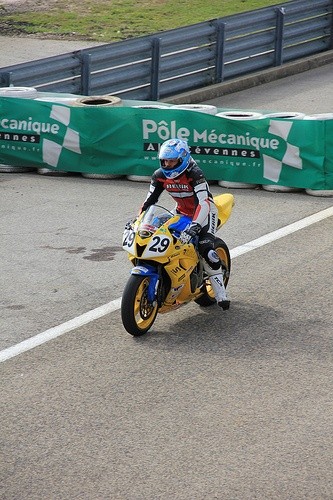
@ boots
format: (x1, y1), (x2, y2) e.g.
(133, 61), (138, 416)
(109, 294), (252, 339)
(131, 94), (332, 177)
(208, 273), (230, 311)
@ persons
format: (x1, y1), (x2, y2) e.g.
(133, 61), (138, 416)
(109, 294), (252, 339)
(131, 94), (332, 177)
(132, 138), (231, 311)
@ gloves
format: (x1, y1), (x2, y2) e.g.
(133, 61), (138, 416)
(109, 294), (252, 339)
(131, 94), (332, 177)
(180, 222), (202, 244)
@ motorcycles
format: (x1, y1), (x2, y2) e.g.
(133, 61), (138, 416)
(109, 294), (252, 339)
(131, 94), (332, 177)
(120, 193), (235, 337)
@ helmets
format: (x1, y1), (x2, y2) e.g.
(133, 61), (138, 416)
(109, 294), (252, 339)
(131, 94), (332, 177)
(158, 139), (192, 180)
